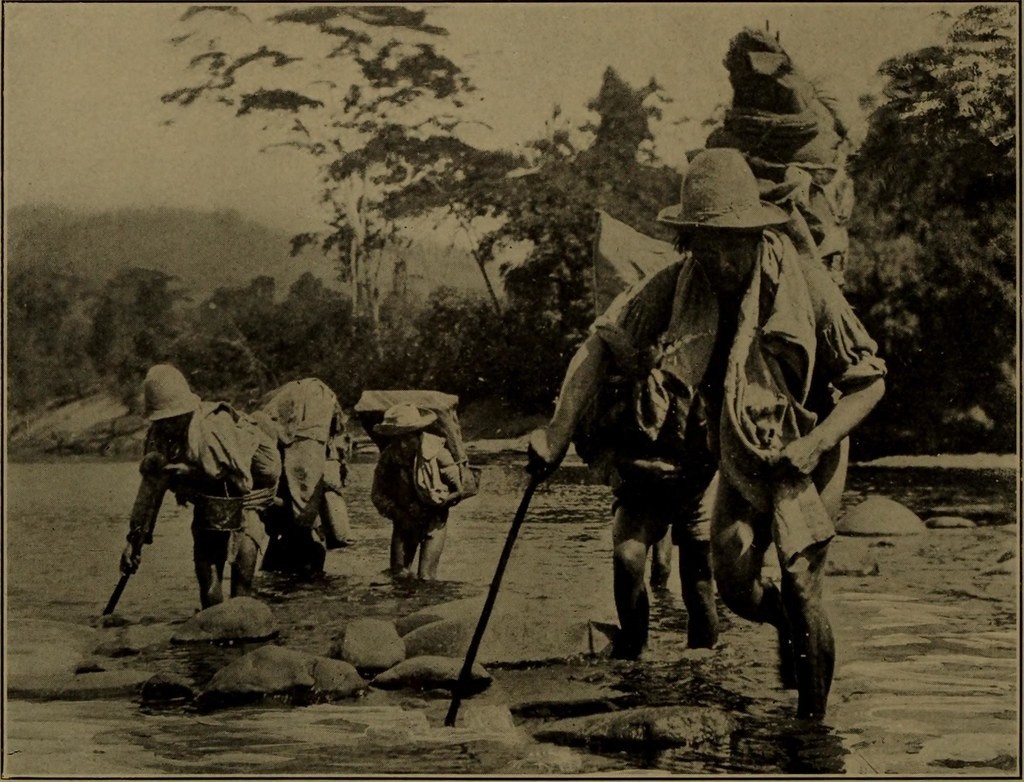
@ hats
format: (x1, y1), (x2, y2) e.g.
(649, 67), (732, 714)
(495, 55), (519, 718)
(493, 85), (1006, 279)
(655, 147), (790, 232)
(141, 364), (200, 422)
(373, 401), (438, 436)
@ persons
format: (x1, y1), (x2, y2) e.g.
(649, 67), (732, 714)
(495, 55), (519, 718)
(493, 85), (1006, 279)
(521, 25), (887, 727)
(118, 364), (477, 610)
